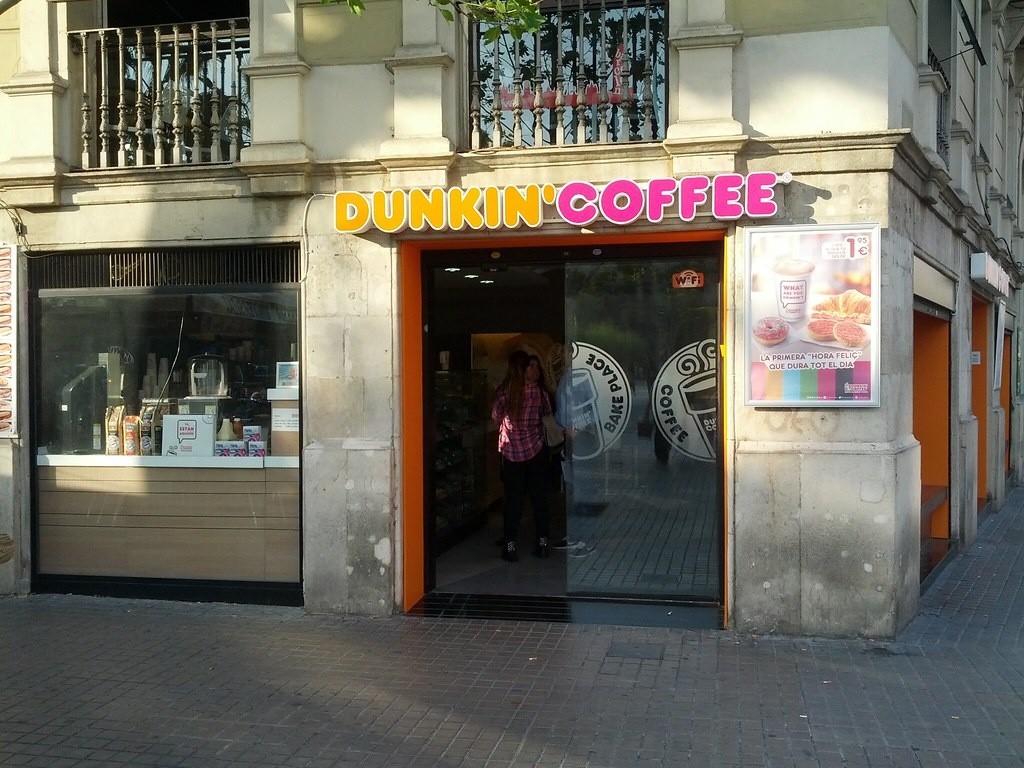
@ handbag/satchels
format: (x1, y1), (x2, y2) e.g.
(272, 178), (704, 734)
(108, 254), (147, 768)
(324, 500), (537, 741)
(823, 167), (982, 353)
(538, 387), (565, 449)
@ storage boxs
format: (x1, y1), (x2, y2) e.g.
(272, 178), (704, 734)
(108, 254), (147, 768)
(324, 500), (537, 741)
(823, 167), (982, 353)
(162, 414), (267, 457)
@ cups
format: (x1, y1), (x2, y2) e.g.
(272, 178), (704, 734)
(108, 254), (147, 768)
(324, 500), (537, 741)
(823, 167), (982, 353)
(774, 261), (815, 323)
(138, 354), (169, 397)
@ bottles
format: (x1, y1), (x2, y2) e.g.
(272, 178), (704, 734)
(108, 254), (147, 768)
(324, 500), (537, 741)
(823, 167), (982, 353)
(216, 416), (236, 441)
(234, 418), (243, 439)
(186, 352), (224, 396)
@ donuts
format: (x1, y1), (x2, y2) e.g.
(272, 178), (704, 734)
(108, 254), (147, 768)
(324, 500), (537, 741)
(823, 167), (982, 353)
(753, 316), (790, 346)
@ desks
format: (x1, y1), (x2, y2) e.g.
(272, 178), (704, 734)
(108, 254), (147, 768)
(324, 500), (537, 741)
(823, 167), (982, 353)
(751, 291), (871, 400)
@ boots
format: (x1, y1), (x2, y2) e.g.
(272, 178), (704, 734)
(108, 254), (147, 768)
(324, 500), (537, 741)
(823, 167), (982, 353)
(534, 535), (552, 559)
(501, 535), (522, 563)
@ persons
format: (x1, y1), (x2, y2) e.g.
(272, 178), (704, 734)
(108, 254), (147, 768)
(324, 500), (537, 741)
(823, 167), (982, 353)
(647, 368), (694, 468)
(490, 349), (596, 562)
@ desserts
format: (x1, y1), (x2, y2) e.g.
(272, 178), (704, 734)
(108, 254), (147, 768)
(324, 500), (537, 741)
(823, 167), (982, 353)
(833, 321), (868, 347)
(807, 319), (839, 341)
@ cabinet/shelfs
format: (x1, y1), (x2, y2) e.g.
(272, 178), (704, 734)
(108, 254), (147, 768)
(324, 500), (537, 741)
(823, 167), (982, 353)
(422, 369), (491, 556)
(268, 387), (300, 457)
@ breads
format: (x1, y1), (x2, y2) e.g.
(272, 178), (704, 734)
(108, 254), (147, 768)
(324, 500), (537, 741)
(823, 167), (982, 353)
(811, 289), (873, 324)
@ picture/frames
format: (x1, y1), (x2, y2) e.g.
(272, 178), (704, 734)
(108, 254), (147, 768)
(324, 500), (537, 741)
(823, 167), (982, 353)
(275, 361), (299, 388)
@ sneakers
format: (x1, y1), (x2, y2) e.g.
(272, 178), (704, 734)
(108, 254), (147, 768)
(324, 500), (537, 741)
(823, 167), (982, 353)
(567, 541), (598, 558)
(551, 534), (580, 549)
